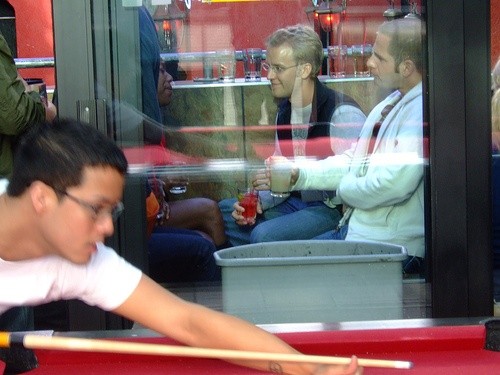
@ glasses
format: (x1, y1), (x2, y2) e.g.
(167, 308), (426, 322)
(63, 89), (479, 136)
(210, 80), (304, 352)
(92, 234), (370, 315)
(261, 62), (303, 74)
(37, 178), (125, 221)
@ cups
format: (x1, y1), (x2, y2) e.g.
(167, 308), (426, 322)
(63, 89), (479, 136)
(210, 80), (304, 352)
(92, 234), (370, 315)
(168, 160), (188, 194)
(238, 187), (258, 223)
(147, 173), (166, 218)
(217, 49), (235, 83)
(269, 162), (291, 198)
(328, 46), (347, 78)
(242, 48), (262, 81)
(351, 44), (370, 77)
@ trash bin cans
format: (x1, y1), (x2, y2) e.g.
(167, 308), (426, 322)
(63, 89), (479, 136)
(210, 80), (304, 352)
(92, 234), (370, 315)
(214, 239), (408, 325)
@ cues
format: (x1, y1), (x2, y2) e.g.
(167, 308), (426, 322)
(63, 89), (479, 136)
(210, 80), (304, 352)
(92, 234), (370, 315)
(0, 332), (412, 369)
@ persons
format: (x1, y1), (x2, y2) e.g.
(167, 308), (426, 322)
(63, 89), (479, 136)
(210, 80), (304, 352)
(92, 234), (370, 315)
(218, 23), (367, 247)
(51, 4), (232, 287)
(228, 0), (318, 126)
(0, 0), (57, 195)
(0, 118), (364, 375)
(266, 17), (423, 279)
